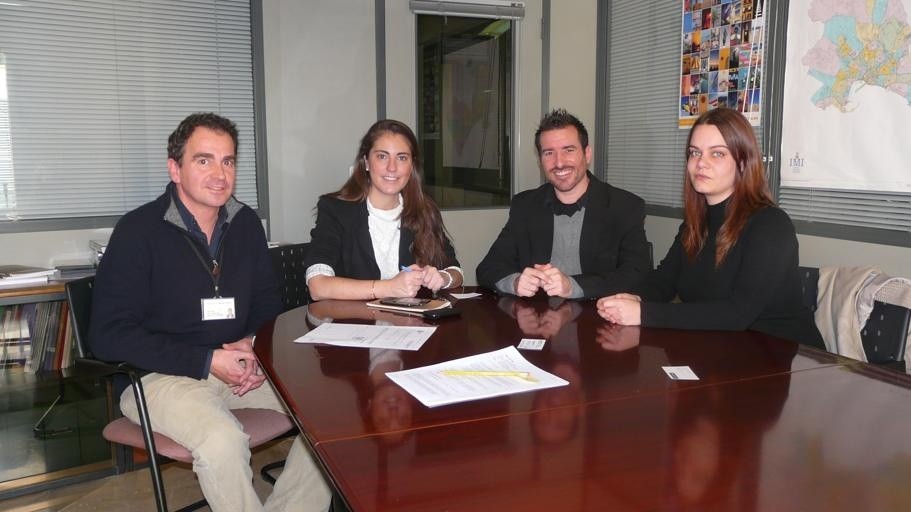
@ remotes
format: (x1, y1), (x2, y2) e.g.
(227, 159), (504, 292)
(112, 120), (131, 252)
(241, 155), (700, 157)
(422, 306), (463, 319)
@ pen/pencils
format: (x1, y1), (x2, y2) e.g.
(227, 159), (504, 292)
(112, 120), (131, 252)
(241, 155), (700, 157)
(401, 265), (413, 272)
(441, 371), (530, 376)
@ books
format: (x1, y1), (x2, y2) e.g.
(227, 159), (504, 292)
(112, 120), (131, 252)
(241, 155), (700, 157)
(1, 261), (59, 285)
(1, 298), (82, 374)
(89, 238), (110, 268)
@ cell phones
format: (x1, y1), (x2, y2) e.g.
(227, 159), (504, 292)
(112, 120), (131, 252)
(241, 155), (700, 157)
(380, 297), (431, 307)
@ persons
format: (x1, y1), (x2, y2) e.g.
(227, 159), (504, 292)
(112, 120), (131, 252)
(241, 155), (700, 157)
(87, 110), (335, 512)
(596, 108), (827, 352)
(305, 120), (466, 302)
(474, 108), (653, 303)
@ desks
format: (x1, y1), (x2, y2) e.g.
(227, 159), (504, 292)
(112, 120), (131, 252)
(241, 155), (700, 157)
(257, 279), (911, 512)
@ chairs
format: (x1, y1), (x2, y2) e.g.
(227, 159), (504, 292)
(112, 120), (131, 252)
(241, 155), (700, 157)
(798, 264), (911, 373)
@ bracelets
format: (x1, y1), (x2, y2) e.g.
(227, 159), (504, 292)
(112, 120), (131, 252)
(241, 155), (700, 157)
(439, 269), (454, 290)
(371, 279), (379, 300)
